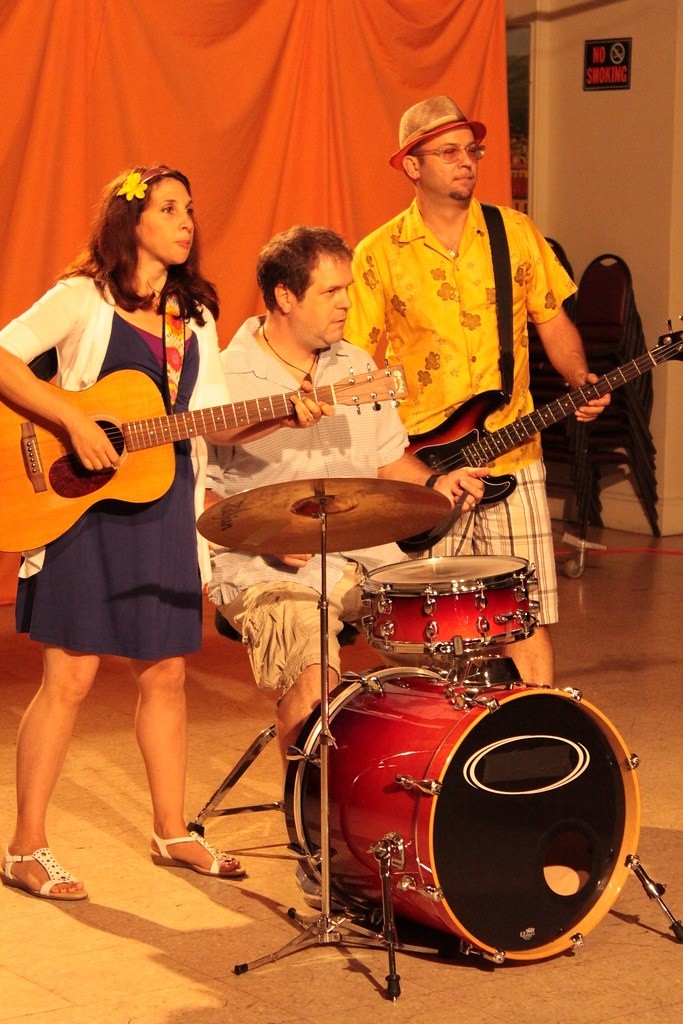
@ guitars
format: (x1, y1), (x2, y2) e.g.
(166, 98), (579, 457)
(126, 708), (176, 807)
(0, 364), (411, 554)
(378, 314), (683, 550)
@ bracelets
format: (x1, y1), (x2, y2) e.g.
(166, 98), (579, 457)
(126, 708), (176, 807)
(425, 473), (447, 488)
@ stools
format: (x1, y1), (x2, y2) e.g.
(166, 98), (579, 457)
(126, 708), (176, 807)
(187, 611), (358, 838)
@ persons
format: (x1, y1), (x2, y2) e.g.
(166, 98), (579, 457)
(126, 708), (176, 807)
(0, 163), (334, 902)
(205, 224), (490, 911)
(341, 95), (612, 689)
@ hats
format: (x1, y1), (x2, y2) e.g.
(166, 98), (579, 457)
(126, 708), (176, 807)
(389, 95), (487, 171)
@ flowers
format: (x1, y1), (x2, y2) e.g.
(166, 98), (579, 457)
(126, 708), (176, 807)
(115, 172), (148, 202)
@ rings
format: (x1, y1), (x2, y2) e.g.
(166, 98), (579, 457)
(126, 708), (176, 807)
(306, 413), (313, 421)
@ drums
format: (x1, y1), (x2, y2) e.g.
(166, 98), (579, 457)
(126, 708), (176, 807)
(364, 555), (542, 654)
(280, 664), (643, 962)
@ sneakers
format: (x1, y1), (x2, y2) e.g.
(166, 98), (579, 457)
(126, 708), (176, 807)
(295, 863), (344, 910)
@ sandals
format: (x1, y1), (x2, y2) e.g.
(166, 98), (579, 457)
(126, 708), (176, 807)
(150, 828), (246, 878)
(0, 846), (88, 901)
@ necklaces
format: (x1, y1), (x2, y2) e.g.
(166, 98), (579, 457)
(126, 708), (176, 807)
(426, 225), (462, 258)
(261, 325), (319, 385)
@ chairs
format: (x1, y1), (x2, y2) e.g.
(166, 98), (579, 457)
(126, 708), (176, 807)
(530, 238), (665, 542)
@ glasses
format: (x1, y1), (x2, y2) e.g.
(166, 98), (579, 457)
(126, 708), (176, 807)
(409, 145), (486, 164)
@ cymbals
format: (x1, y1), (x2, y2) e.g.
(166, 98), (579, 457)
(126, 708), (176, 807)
(195, 479), (453, 553)
